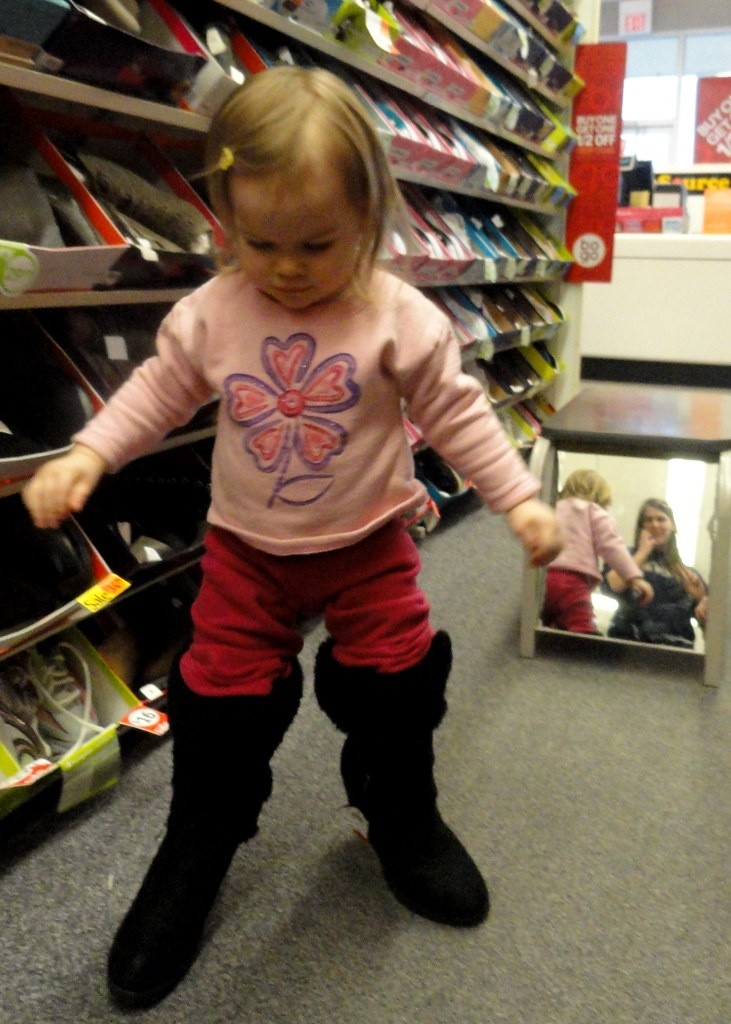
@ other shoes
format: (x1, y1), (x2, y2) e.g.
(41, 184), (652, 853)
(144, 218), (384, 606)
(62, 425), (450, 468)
(0, 0), (256, 789)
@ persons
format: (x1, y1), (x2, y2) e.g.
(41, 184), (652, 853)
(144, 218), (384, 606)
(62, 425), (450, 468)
(544, 467), (656, 635)
(601, 496), (711, 652)
(19, 68), (562, 1015)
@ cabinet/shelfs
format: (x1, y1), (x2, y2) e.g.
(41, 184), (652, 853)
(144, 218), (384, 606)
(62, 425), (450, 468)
(0, 0), (590, 856)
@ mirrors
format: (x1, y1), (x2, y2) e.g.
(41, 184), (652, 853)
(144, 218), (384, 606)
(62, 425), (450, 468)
(517, 427), (731, 687)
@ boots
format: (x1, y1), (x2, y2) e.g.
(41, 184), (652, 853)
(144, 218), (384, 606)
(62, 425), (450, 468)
(316, 623), (489, 927)
(108, 649), (304, 1016)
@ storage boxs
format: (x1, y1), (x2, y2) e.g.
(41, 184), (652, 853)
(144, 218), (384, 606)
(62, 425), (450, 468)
(2, 0), (580, 816)
